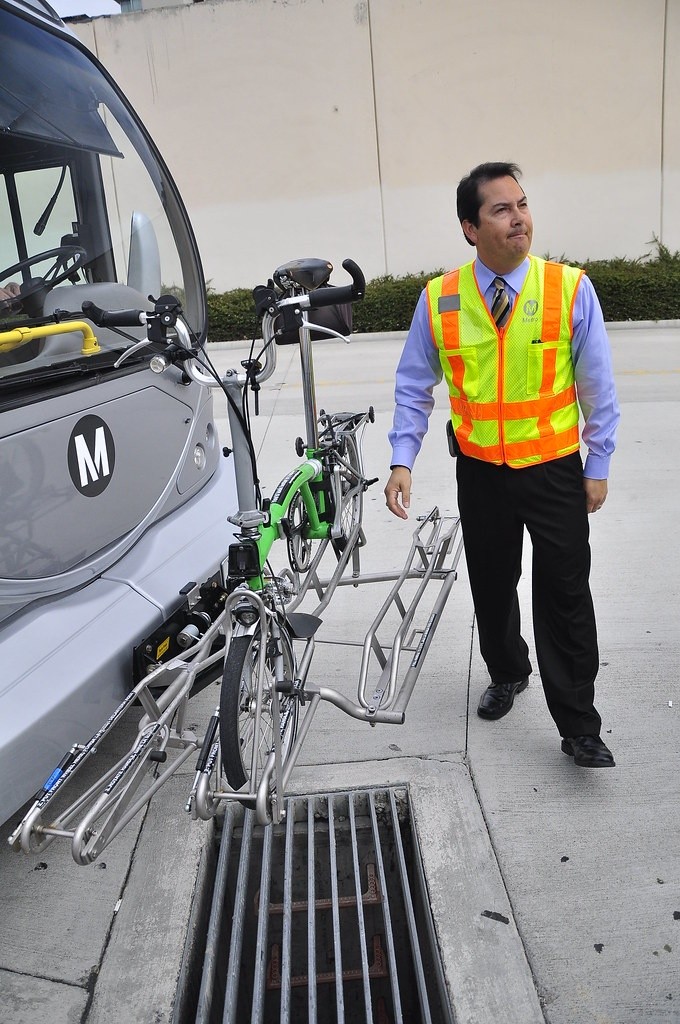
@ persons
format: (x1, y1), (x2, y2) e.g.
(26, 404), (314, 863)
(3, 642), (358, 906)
(385, 162), (616, 769)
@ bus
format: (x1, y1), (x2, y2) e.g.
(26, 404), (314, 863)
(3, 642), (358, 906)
(0, 0), (242, 830)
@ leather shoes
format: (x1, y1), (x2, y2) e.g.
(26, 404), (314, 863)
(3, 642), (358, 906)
(561, 735), (616, 767)
(477, 676), (528, 720)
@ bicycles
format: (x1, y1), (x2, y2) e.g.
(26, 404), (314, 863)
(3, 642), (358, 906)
(80, 257), (381, 813)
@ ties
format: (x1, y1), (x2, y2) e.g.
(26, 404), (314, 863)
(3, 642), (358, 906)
(492, 276), (512, 332)
